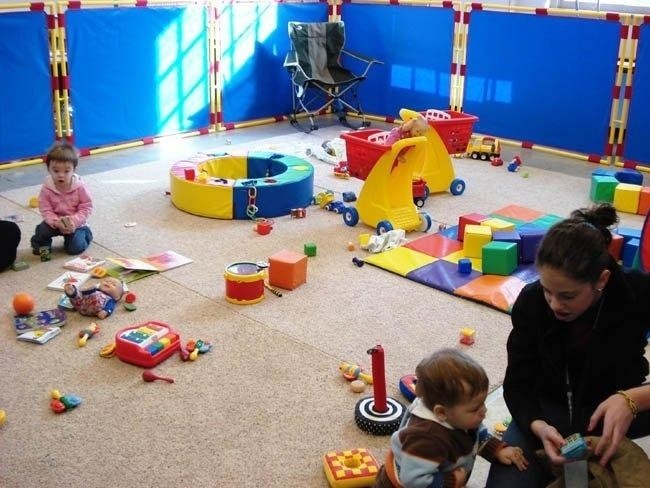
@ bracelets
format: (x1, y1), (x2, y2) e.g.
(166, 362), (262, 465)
(618, 389), (638, 418)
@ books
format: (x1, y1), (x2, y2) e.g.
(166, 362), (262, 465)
(107, 250), (191, 286)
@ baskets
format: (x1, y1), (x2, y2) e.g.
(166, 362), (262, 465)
(340, 129), (407, 181)
(418, 110), (479, 154)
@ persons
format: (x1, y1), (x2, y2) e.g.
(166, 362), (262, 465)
(483, 201), (650, 488)
(32, 141), (93, 255)
(375, 349), (528, 488)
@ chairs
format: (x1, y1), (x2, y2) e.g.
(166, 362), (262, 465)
(283, 21), (385, 133)
